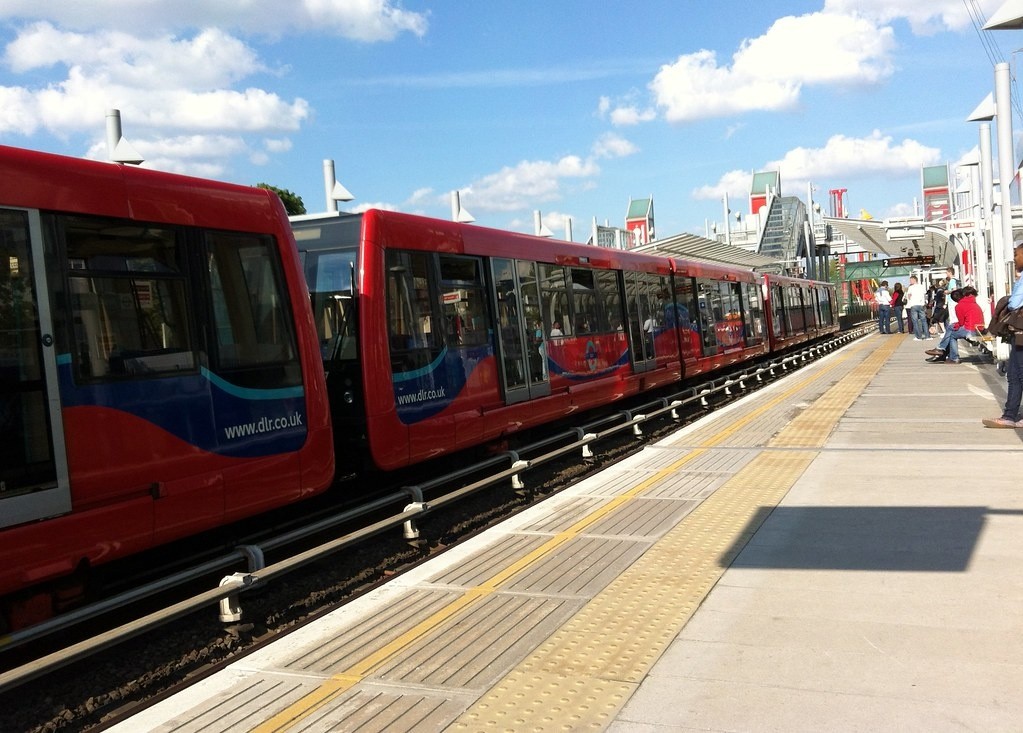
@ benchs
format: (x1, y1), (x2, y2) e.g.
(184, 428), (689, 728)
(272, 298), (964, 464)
(969, 324), (997, 357)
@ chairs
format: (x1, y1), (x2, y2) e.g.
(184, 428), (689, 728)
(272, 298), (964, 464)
(107, 344), (196, 373)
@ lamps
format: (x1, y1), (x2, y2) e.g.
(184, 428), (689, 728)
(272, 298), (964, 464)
(908, 249), (914, 256)
(900, 246), (907, 252)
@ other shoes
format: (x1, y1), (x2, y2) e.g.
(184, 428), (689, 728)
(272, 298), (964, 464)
(1014, 418), (1023, 428)
(924, 355), (946, 363)
(913, 337), (923, 341)
(926, 337), (935, 340)
(925, 347), (943, 355)
(982, 416), (1016, 429)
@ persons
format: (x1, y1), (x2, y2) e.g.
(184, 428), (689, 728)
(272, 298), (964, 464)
(535, 320), (547, 381)
(982, 242), (1023, 429)
(390, 330), (415, 372)
(890, 283), (904, 333)
(643, 311), (658, 334)
(550, 321), (563, 336)
(875, 281), (894, 334)
(908, 276), (933, 341)
(924, 286), (992, 363)
(902, 283), (913, 334)
(926, 267), (960, 338)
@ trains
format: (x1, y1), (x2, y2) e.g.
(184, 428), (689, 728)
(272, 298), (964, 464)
(0, 144), (839, 597)
